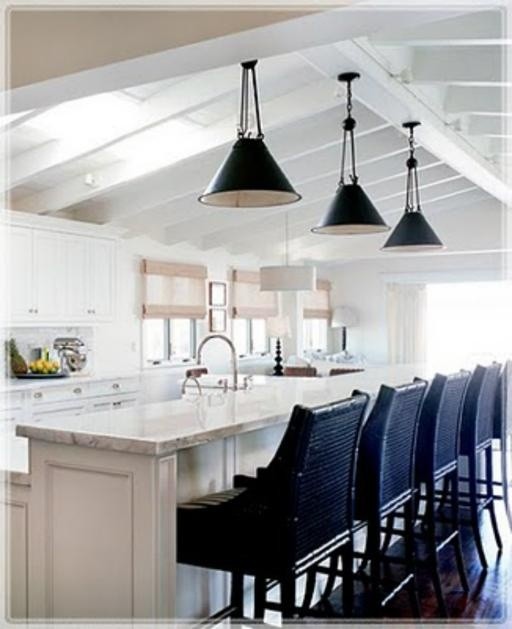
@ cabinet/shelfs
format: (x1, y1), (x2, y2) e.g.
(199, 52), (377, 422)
(27, 378), (90, 423)
(2, 207), (66, 330)
(89, 372), (143, 411)
(68, 219), (120, 327)
(1, 383), (26, 433)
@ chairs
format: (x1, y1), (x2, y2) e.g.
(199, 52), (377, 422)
(329, 368), (365, 376)
(176, 388), (369, 627)
(413, 360), (511, 569)
(284, 367), (316, 377)
(354, 369), (472, 608)
(299, 376), (430, 624)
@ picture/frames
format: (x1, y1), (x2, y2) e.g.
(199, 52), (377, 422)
(210, 308), (227, 332)
(209, 281), (226, 307)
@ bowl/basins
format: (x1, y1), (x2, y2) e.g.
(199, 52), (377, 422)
(66, 354), (87, 371)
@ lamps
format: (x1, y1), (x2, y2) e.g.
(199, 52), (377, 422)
(197, 61), (302, 208)
(266, 315), (293, 376)
(310, 72), (392, 236)
(331, 306), (360, 350)
(379, 122), (446, 254)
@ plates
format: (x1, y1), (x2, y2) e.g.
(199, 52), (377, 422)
(16, 372), (67, 380)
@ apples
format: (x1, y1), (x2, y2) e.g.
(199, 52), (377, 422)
(30, 359), (60, 374)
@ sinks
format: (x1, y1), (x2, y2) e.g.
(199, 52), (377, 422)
(185, 385), (234, 390)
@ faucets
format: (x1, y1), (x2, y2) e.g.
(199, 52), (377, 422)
(197, 334), (238, 392)
(180, 376), (203, 396)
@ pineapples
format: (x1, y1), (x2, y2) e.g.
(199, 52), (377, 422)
(9, 338), (27, 374)
